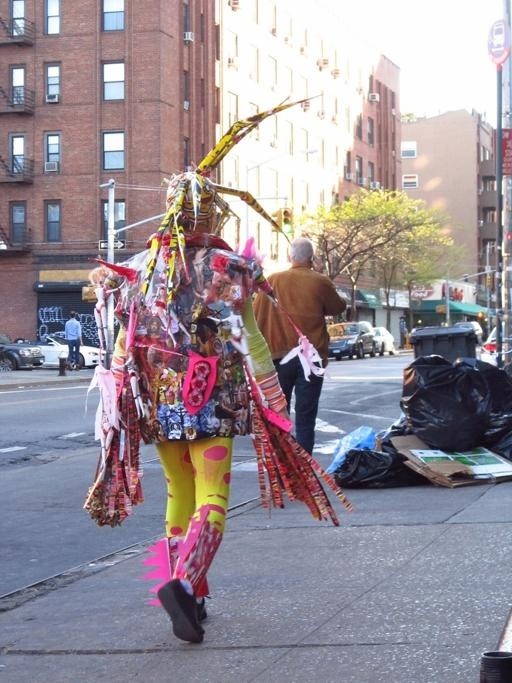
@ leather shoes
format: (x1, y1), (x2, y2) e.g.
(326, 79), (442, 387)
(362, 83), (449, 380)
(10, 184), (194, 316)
(67, 361), (81, 370)
(158, 578), (207, 642)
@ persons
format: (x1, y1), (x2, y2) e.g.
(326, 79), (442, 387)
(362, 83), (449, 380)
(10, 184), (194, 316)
(253, 237), (346, 486)
(94, 173), (347, 641)
(65, 310), (84, 372)
(399, 317), (408, 350)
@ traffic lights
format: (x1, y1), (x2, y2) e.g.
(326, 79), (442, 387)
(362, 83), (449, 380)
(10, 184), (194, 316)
(271, 208), (293, 233)
(486, 277), (490, 288)
(449, 288), (463, 301)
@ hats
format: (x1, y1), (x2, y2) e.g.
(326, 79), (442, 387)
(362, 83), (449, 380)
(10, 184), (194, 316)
(165, 171), (216, 223)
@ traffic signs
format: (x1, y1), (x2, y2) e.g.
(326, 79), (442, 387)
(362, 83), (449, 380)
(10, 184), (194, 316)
(99, 239), (126, 251)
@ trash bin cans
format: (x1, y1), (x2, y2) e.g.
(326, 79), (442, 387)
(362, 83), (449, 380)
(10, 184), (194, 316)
(410, 328), (477, 364)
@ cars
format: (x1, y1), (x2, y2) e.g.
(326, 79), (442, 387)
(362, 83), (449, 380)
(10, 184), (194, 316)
(454, 321), (511, 365)
(327, 321), (394, 360)
(0, 335), (106, 372)
